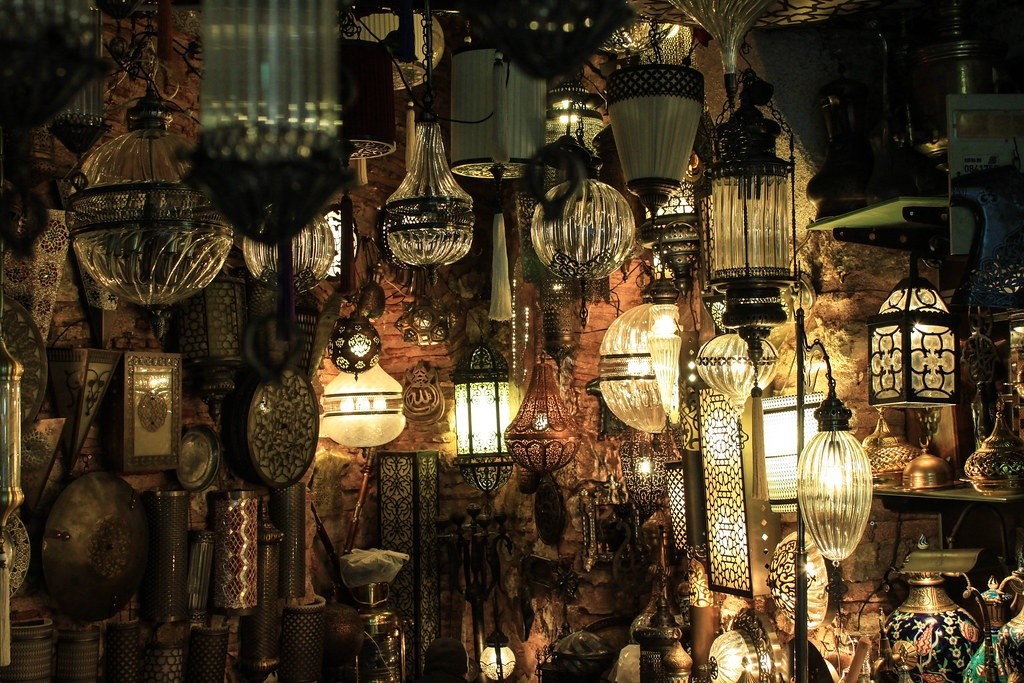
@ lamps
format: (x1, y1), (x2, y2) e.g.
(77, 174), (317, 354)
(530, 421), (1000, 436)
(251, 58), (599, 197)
(0, 0), (1024, 683)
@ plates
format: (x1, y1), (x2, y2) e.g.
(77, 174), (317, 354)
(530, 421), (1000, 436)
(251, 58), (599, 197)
(176, 423), (218, 491)
